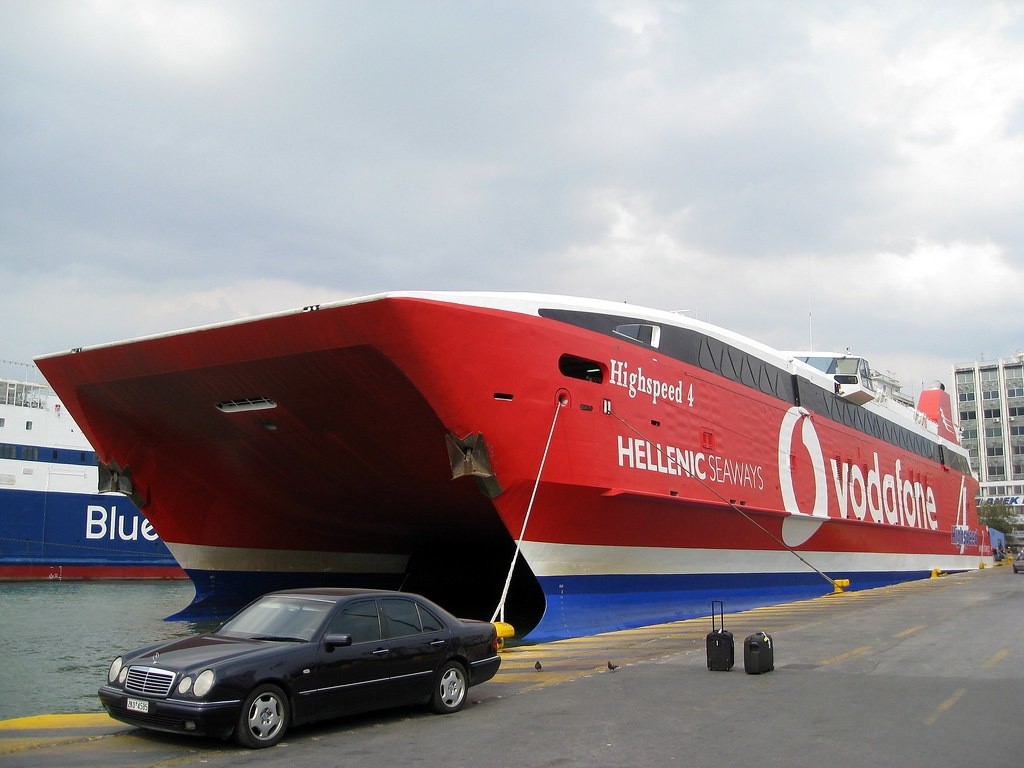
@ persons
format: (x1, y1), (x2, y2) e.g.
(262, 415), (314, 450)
(993, 544), (1013, 561)
(835, 384), (841, 392)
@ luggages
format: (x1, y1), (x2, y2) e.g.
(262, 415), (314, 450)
(706, 601), (734, 671)
(744, 633), (774, 674)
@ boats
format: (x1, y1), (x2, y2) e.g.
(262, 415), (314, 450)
(30, 283), (1007, 650)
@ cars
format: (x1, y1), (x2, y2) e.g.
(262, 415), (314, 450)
(1012, 552), (1024, 573)
(99, 585), (503, 749)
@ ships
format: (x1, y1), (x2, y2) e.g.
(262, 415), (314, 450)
(0, 375), (193, 584)
